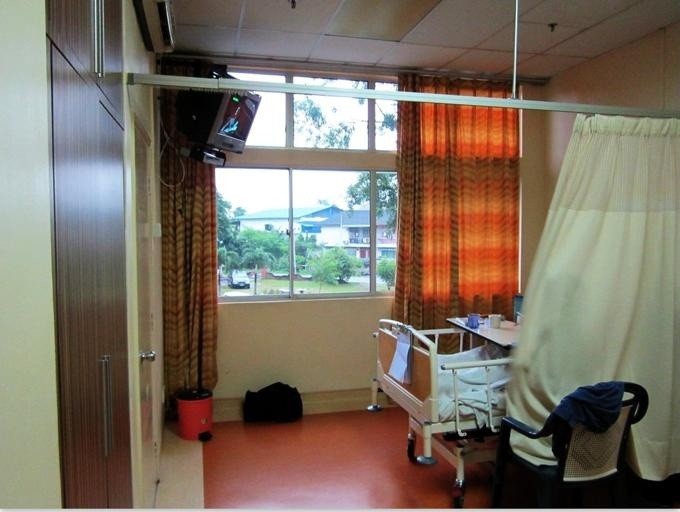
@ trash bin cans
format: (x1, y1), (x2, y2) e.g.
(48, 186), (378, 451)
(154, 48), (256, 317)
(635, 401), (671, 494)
(175, 387), (213, 440)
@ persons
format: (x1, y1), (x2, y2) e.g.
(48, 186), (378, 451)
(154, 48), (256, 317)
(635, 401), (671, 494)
(221, 107), (241, 137)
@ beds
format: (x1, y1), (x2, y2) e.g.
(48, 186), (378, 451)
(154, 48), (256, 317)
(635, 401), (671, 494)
(367, 319), (507, 491)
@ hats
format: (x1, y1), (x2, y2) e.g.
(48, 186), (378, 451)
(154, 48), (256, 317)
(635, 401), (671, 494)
(236, 106), (241, 114)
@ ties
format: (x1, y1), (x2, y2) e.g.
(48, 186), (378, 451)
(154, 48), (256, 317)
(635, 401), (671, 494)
(230, 119), (237, 127)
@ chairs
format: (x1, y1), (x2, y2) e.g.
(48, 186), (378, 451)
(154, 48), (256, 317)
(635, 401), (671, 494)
(493, 380), (649, 509)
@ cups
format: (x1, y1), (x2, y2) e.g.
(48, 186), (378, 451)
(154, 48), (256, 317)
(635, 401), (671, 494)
(467, 313), (480, 328)
(489, 315), (501, 329)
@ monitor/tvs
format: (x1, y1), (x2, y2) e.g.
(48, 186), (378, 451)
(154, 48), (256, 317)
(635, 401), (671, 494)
(175, 65), (262, 155)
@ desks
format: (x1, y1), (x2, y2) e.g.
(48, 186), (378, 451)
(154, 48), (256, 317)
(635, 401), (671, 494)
(445, 316), (520, 347)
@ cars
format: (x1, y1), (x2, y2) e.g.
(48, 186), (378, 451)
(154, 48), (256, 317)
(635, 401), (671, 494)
(227, 270), (251, 289)
